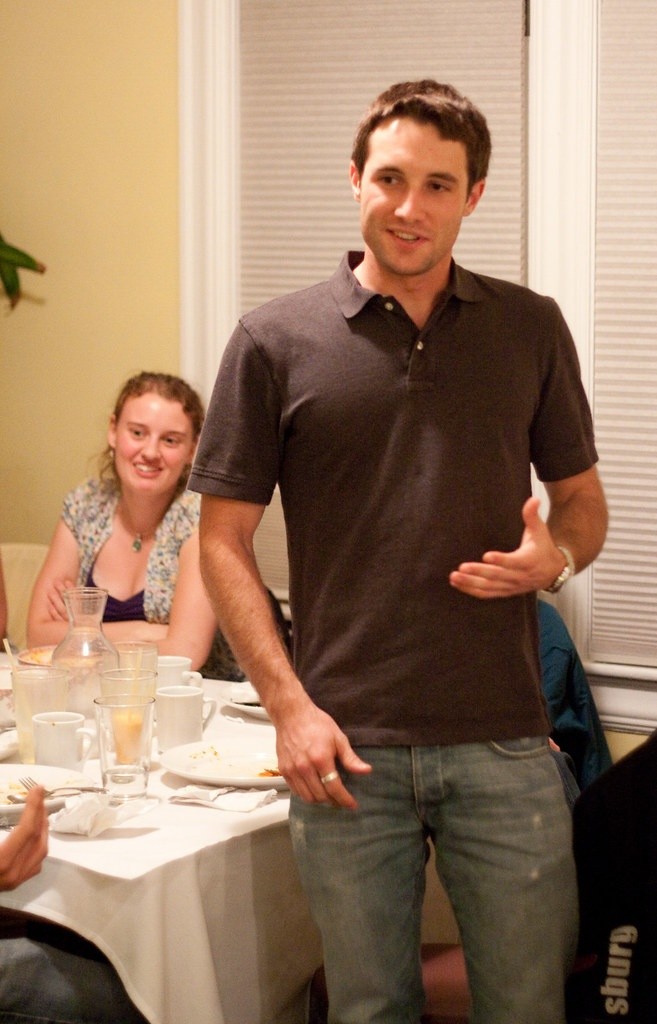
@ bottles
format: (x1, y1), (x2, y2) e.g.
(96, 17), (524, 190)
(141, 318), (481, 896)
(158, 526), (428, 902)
(52, 587), (120, 759)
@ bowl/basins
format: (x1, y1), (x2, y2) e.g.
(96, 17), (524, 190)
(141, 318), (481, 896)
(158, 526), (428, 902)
(17, 645), (58, 666)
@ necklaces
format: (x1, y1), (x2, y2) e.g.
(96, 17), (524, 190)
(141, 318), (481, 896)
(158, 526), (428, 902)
(132, 531), (148, 552)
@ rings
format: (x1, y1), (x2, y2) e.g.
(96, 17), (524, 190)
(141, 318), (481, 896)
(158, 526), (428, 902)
(321, 770), (338, 784)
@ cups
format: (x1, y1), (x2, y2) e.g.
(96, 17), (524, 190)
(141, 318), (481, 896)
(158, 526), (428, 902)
(100, 669), (157, 696)
(32, 712), (98, 770)
(10, 667), (69, 763)
(94, 695), (155, 796)
(156, 686), (217, 751)
(112, 640), (158, 670)
(157, 654), (203, 688)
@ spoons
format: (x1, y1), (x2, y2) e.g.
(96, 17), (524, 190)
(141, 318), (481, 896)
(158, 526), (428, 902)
(6, 792), (81, 804)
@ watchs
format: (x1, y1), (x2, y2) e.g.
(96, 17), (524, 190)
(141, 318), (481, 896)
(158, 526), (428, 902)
(541, 546), (575, 595)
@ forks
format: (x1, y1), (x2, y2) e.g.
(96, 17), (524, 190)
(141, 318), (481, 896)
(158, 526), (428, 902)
(19, 776), (107, 794)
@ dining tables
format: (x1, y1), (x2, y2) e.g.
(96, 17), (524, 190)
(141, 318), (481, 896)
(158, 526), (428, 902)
(1, 648), (324, 1024)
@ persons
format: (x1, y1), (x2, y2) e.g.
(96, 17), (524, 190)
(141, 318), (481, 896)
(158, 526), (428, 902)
(25, 372), (245, 682)
(0, 784), (149, 1024)
(186, 80), (610, 1024)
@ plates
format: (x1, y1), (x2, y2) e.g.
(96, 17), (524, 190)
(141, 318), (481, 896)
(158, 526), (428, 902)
(158, 738), (289, 792)
(0, 763), (95, 826)
(219, 686), (271, 721)
